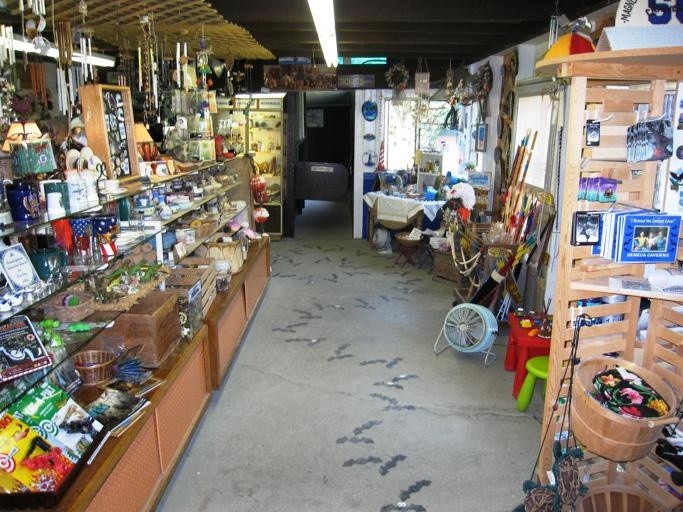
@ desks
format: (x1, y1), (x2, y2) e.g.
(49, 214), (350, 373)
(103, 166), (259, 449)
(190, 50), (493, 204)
(362, 189), (453, 250)
(504, 311), (553, 400)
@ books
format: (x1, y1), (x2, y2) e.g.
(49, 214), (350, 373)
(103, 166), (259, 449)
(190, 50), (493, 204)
(109, 376), (167, 396)
(83, 386), (151, 435)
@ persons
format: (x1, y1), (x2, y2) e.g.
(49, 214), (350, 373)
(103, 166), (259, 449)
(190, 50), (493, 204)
(633, 232), (646, 250)
(647, 233), (655, 249)
(655, 231), (666, 250)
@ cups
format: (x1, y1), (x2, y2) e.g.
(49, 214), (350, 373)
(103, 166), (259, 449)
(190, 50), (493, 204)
(69, 216), (93, 259)
(39, 176), (98, 212)
(51, 218), (73, 257)
(91, 214), (117, 256)
(424, 191), (435, 201)
(27, 247), (66, 280)
(183, 228), (195, 244)
(138, 162), (153, 178)
(103, 178), (119, 192)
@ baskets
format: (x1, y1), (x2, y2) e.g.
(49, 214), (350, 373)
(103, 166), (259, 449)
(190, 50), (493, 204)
(159, 257), (216, 320)
(43, 292), (94, 322)
(72, 350), (114, 386)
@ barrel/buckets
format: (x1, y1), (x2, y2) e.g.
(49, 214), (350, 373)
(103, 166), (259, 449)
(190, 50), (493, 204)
(570, 355), (680, 462)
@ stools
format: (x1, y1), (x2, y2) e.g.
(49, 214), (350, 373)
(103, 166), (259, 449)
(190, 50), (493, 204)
(419, 239), (435, 274)
(515, 355), (549, 412)
(390, 244), (418, 268)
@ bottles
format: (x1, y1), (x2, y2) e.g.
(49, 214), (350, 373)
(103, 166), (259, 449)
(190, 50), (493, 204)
(426, 159), (439, 174)
(152, 190), (169, 220)
(4, 182), (38, 222)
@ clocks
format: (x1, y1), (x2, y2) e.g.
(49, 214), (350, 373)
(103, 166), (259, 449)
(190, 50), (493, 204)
(306, 108), (325, 128)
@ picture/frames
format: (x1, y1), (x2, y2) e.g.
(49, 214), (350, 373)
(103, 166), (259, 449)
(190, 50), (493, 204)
(467, 171), (497, 216)
(151, 162), (171, 177)
(475, 123), (488, 152)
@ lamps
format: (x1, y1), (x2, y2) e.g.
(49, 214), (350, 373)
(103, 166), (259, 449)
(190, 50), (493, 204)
(0, 0), (116, 68)
(1, 122), (42, 153)
(535, 17), (597, 74)
(134, 121), (154, 143)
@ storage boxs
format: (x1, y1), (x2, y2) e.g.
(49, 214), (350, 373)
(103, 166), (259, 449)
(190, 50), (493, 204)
(43, 256), (217, 367)
(429, 249), (463, 289)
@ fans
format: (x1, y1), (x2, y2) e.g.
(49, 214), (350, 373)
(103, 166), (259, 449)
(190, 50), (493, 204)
(434, 303), (498, 367)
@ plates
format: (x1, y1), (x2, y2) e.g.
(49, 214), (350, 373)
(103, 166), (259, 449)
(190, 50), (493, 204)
(99, 188), (127, 195)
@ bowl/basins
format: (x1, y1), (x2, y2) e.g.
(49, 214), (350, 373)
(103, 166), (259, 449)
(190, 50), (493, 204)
(394, 232), (423, 247)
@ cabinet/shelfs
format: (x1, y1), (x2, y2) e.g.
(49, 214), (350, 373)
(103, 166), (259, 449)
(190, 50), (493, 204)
(80, 84), (142, 185)
(532, 46), (683, 512)
(417, 152), (442, 193)
(211, 92), (296, 241)
(0, 154), (272, 512)
